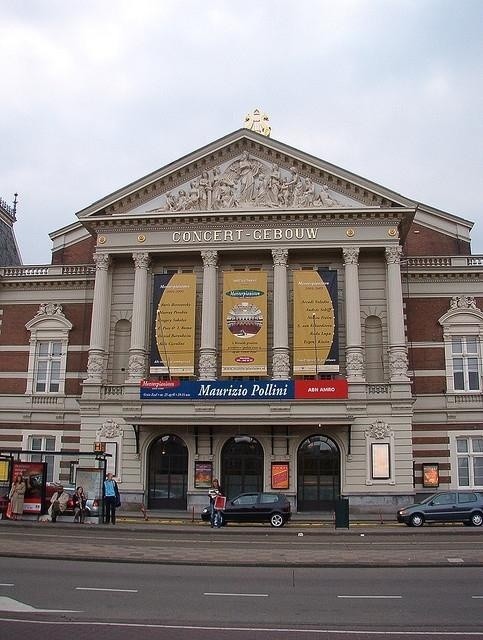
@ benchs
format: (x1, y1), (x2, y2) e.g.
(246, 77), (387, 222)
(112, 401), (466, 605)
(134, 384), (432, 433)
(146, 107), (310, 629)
(51, 510), (91, 523)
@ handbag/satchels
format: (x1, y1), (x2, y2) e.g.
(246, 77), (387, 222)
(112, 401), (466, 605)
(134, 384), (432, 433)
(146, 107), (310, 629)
(115, 494), (121, 507)
(6, 502), (13, 519)
(214, 496), (226, 510)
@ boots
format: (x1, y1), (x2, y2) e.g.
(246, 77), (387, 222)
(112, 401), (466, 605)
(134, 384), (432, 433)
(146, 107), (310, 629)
(211, 522), (221, 528)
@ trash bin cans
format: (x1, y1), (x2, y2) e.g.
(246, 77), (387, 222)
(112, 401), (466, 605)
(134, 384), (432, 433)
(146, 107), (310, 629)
(335, 499), (349, 530)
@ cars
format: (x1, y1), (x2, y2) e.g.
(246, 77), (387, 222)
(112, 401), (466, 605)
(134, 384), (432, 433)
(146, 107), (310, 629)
(150, 489), (176, 499)
(0, 482), (106, 517)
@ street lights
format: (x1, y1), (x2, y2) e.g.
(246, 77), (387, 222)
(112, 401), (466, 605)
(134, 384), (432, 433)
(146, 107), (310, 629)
(160, 449), (171, 501)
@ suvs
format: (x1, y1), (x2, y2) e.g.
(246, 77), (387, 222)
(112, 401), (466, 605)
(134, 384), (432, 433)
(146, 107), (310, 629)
(200, 490), (293, 528)
(396, 489), (482, 528)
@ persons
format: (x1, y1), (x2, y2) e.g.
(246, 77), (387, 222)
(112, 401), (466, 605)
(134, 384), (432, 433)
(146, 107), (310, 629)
(48, 485), (69, 522)
(144, 151), (336, 212)
(208, 479), (224, 528)
(8, 474), (26, 520)
(72, 487), (88, 524)
(104, 473), (118, 525)
